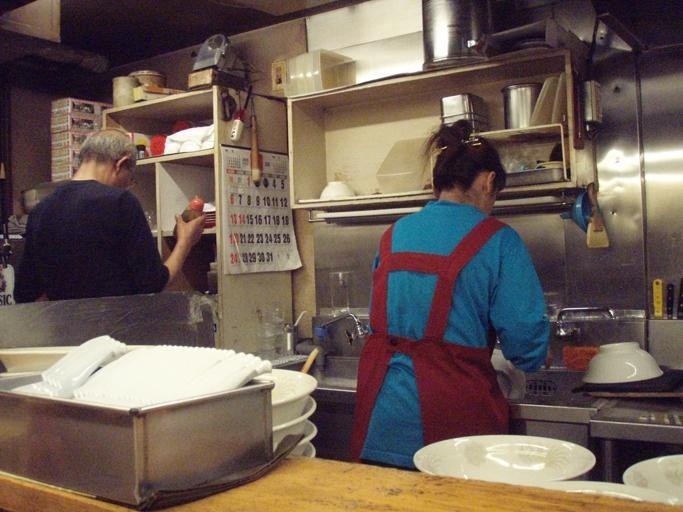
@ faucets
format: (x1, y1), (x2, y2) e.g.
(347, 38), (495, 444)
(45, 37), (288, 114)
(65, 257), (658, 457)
(282, 311), (308, 354)
(554, 304), (619, 341)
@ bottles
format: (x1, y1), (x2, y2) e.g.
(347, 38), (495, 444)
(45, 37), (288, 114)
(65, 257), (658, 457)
(136, 145), (145, 160)
(173, 196), (204, 237)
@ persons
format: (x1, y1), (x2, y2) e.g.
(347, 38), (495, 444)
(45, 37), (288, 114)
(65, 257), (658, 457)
(345, 118), (553, 474)
(12, 126), (207, 308)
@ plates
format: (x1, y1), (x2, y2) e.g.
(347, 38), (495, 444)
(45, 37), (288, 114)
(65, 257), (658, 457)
(202, 197), (217, 228)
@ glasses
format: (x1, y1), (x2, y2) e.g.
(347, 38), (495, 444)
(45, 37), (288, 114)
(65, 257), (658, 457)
(124, 164), (137, 189)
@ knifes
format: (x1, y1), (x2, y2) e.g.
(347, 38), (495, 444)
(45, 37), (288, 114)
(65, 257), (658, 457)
(653, 278), (682, 320)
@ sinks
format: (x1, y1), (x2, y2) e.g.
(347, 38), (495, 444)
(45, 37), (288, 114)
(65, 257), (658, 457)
(274, 354), (360, 391)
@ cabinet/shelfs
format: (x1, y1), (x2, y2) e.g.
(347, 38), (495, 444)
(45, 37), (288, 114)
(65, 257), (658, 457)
(287, 49), (599, 224)
(100, 85), (293, 356)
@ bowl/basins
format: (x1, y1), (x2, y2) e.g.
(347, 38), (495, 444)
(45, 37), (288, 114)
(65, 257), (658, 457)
(579, 342), (662, 386)
(319, 180), (355, 201)
(21, 187), (40, 214)
(411, 424), (683, 506)
(254, 368), (319, 456)
